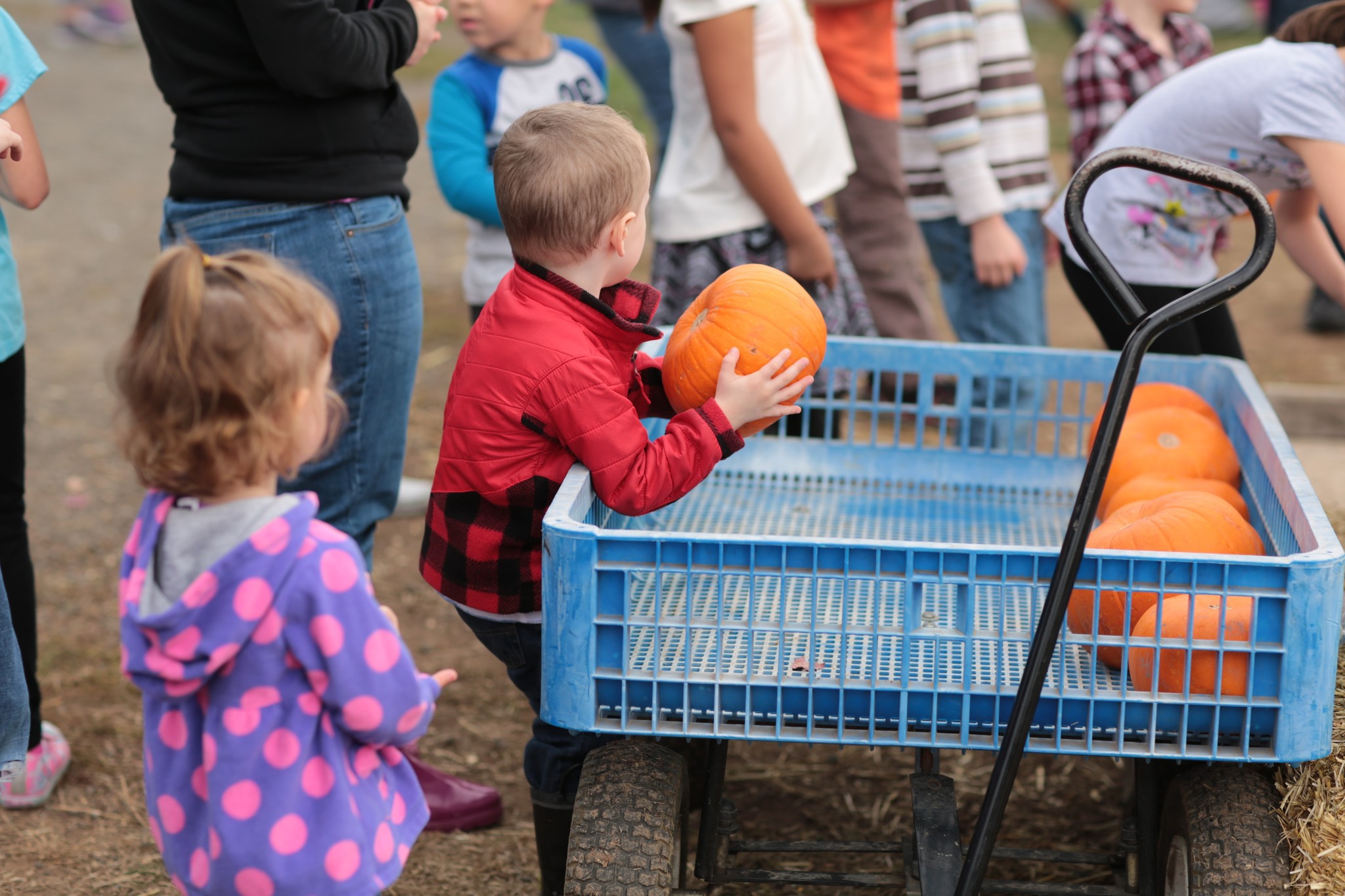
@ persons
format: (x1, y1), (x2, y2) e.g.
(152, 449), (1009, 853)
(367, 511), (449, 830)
(418, 104), (814, 896)
(1041, 0), (1345, 363)
(893, 0), (1058, 448)
(133, 0), (503, 834)
(426, 0), (610, 328)
(0, 0), (71, 810)
(120, 244), (457, 896)
(587, 0), (966, 441)
(1062, 0), (1211, 172)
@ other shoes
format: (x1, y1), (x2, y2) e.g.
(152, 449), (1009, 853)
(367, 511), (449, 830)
(393, 476), (433, 518)
(396, 752), (505, 833)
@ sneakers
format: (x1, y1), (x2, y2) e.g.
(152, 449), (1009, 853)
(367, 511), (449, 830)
(0, 719), (71, 810)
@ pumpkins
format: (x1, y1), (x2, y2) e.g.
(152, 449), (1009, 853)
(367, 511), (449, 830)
(1065, 491), (1265, 669)
(1127, 594), (1252, 696)
(1096, 407), (1240, 520)
(1103, 475), (1251, 524)
(1088, 382), (1223, 457)
(661, 264), (827, 438)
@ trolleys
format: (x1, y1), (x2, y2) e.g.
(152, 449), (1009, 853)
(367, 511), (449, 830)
(538, 141), (1345, 896)
